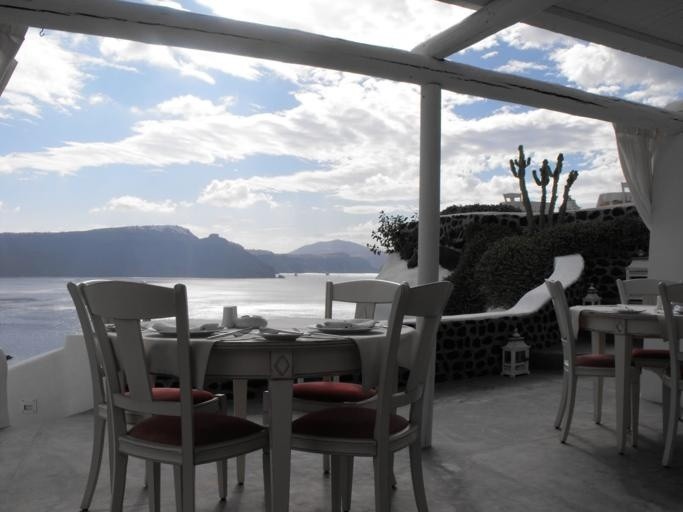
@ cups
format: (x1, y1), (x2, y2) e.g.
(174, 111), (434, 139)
(223, 306), (238, 328)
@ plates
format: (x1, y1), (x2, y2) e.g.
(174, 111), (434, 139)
(261, 332), (302, 342)
(316, 321), (371, 334)
(138, 316), (223, 336)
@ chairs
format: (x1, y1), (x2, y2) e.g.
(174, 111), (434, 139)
(546, 277), (683, 471)
(65, 278), (268, 512)
(292, 278), (455, 512)
